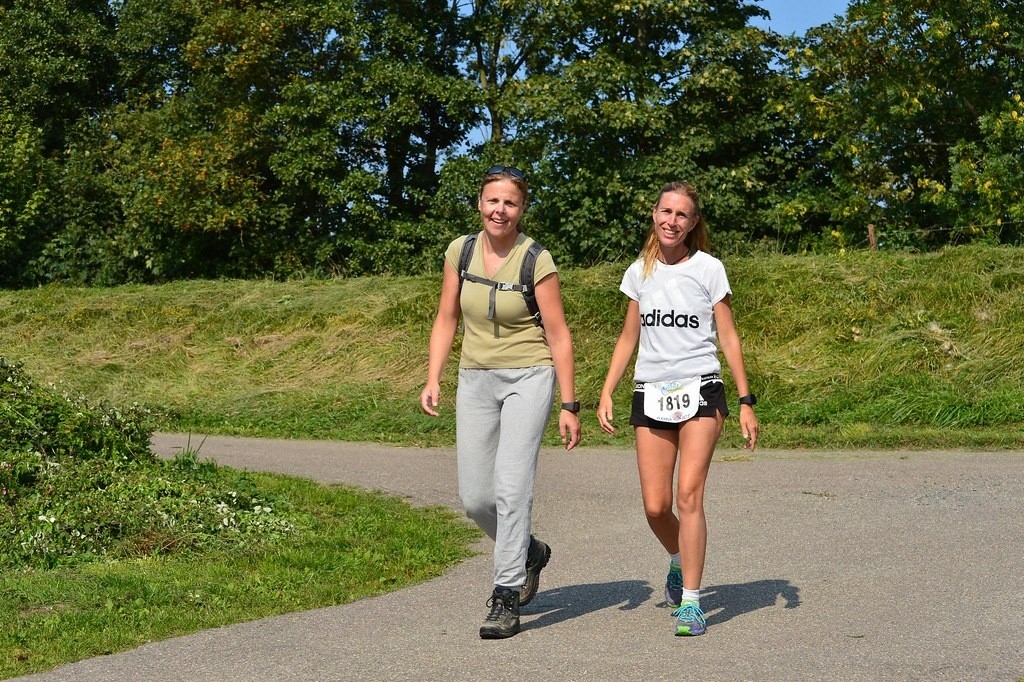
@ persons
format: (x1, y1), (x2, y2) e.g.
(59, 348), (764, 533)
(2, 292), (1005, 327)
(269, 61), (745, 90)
(596, 182), (761, 636)
(419, 166), (584, 639)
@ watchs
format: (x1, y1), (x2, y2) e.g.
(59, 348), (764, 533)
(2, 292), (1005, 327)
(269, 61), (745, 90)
(738, 394), (756, 405)
(558, 401), (580, 413)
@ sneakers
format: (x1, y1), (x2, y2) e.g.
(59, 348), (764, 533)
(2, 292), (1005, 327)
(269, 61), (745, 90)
(479, 587), (521, 637)
(672, 599), (707, 636)
(665, 560), (684, 607)
(518, 534), (551, 606)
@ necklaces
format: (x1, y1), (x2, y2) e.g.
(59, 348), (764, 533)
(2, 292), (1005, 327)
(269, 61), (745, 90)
(484, 231), (518, 269)
(658, 248), (690, 265)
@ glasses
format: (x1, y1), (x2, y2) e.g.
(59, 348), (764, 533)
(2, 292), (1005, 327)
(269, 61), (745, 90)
(485, 165), (527, 180)
